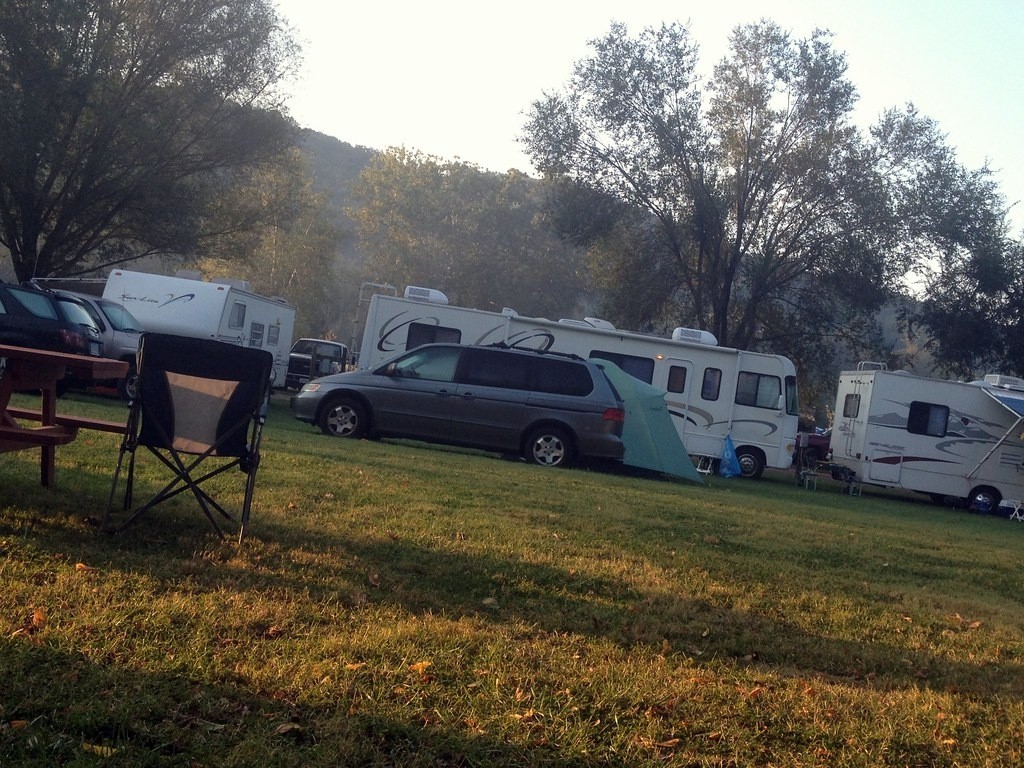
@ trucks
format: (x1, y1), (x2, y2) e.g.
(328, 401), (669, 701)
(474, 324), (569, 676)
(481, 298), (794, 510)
(357, 285), (801, 482)
(826, 363), (1023, 517)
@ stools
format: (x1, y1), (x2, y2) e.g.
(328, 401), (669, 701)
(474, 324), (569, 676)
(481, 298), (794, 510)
(1009, 499), (1024, 522)
(804, 472), (819, 490)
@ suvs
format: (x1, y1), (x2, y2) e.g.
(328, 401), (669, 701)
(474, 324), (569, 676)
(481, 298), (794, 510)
(292, 343), (628, 473)
(794, 427), (832, 470)
(49, 289), (149, 402)
(288, 337), (348, 394)
(0, 280), (106, 401)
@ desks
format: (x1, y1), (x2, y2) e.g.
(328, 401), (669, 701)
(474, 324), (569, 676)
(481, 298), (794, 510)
(0, 406), (130, 447)
(0, 344), (128, 487)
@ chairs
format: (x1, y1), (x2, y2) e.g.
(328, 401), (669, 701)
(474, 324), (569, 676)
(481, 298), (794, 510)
(305, 347), (313, 353)
(100, 332), (273, 547)
